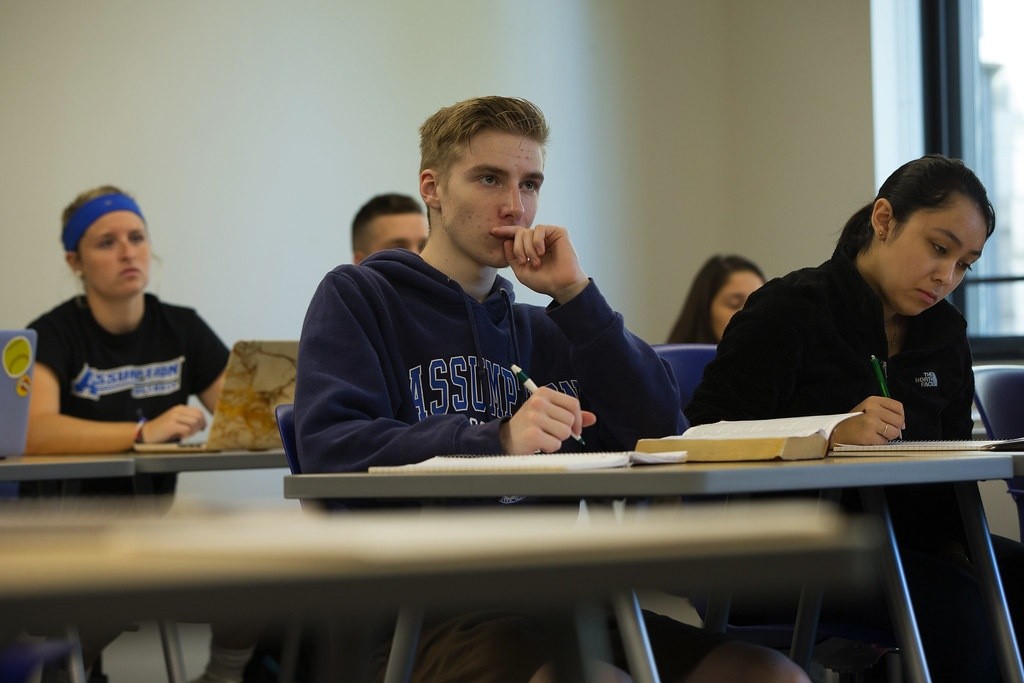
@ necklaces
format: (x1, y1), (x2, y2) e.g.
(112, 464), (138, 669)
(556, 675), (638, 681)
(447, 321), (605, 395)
(890, 314), (898, 348)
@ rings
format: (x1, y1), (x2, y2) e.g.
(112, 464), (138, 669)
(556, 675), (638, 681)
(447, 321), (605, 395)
(882, 424), (888, 436)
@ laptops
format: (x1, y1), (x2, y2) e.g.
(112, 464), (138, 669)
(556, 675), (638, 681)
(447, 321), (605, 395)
(0, 329), (37, 460)
(135, 338), (304, 453)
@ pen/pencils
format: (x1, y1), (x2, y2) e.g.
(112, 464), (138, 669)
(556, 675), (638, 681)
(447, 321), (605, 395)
(510, 363), (586, 445)
(871, 355), (904, 440)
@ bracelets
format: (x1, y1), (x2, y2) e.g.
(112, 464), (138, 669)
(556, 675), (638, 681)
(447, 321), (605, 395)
(134, 416), (147, 445)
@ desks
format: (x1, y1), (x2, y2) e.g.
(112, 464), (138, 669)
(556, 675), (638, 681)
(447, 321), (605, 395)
(0, 436), (1024, 683)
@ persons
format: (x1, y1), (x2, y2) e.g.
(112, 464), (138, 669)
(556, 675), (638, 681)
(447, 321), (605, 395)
(293, 94), (808, 680)
(664, 253), (766, 345)
(684, 155), (1023, 681)
(352, 193), (429, 266)
(20, 184), (269, 683)
